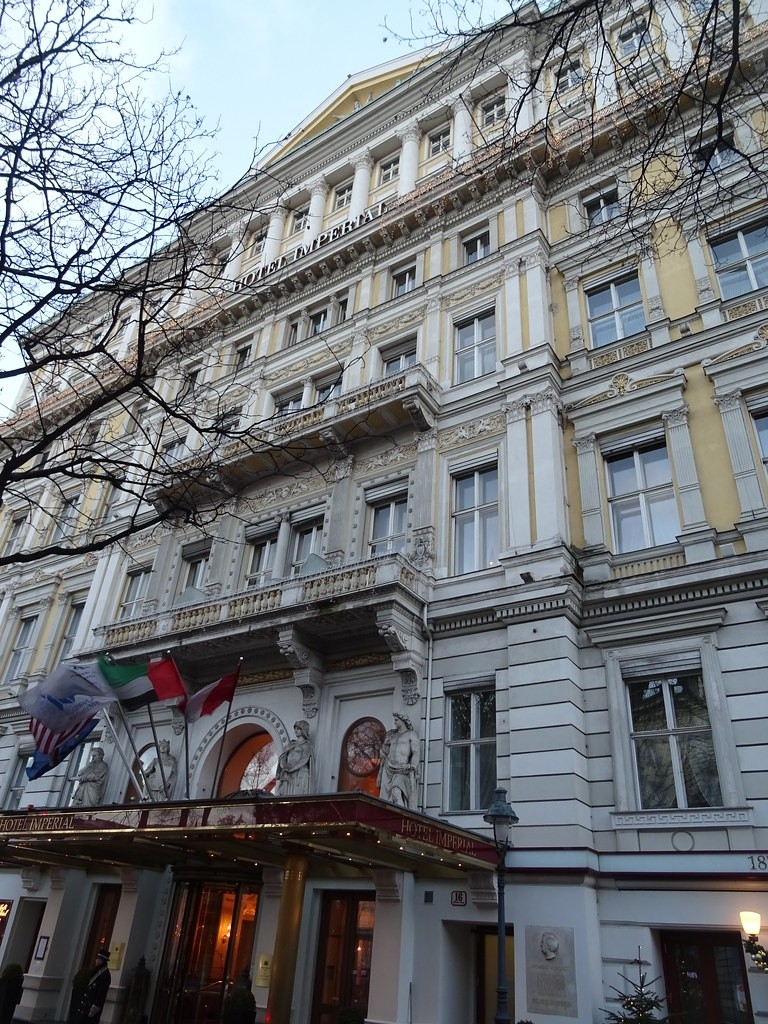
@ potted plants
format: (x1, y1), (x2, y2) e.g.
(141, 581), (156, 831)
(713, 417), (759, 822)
(222, 988), (257, 1024)
(0, 963), (24, 1024)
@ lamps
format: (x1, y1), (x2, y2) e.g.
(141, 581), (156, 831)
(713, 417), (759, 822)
(740, 909), (765, 953)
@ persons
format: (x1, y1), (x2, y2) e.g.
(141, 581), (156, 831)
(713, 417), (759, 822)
(274, 720), (317, 795)
(377, 711), (421, 808)
(141, 739), (179, 802)
(73, 949), (111, 1023)
(71, 747), (109, 807)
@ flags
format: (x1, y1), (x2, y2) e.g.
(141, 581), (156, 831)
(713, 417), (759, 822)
(17, 653), (239, 781)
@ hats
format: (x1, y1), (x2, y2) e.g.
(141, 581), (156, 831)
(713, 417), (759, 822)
(96, 949), (110, 961)
